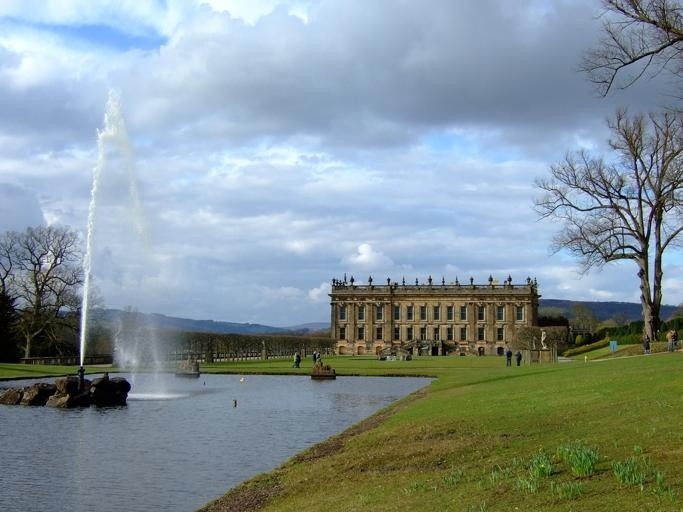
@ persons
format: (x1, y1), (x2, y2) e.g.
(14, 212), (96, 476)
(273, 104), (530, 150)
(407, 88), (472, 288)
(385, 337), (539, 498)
(313, 351), (320, 368)
(506, 348), (512, 367)
(292, 352), (298, 367)
(665, 328), (678, 352)
(311, 350), (316, 366)
(295, 354), (300, 368)
(643, 334), (650, 353)
(539, 328), (549, 350)
(514, 350), (521, 366)
